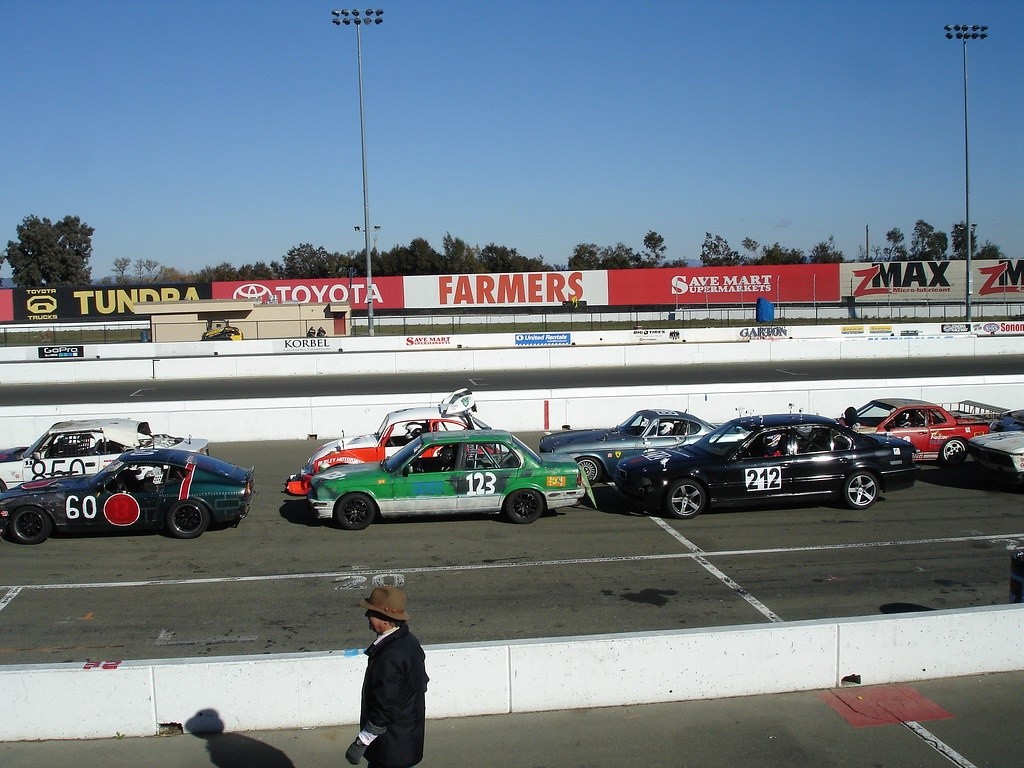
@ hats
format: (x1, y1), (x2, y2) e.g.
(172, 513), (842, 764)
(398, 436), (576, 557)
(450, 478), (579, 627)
(360, 587), (410, 621)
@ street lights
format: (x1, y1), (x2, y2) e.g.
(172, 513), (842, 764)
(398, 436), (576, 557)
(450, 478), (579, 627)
(955, 222), (978, 259)
(354, 223), (381, 276)
(944, 24), (989, 323)
(332, 7), (386, 336)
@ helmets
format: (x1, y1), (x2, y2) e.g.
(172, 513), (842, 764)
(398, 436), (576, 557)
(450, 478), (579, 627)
(136, 466), (153, 481)
(405, 423), (422, 439)
(764, 434), (781, 448)
(901, 413), (914, 427)
(438, 449), (456, 466)
(659, 422), (674, 434)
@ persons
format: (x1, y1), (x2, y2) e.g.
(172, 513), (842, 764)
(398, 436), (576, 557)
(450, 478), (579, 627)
(405, 423), (423, 442)
(897, 412), (911, 427)
(763, 434), (781, 457)
(307, 327), (325, 338)
(132, 466), (155, 494)
(437, 446), (455, 471)
(346, 585), (429, 768)
(659, 422), (674, 436)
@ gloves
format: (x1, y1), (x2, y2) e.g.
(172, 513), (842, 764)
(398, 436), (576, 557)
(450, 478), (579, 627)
(345, 740), (367, 765)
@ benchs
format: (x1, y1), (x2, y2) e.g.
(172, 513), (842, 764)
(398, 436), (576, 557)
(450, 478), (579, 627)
(803, 427), (849, 452)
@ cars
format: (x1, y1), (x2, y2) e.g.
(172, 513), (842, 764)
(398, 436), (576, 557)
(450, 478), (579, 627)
(833, 398), (989, 468)
(202, 326), (242, 342)
(280, 406), (512, 496)
(0, 448), (256, 545)
(614, 415), (922, 520)
(538, 408), (718, 486)
(966, 430), (1024, 490)
(988, 409), (1024, 432)
(0, 418), (210, 495)
(307, 430), (586, 531)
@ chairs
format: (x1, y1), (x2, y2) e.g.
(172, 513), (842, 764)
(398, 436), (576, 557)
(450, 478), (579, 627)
(924, 411), (933, 425)
(907, 411), (918, 427)
